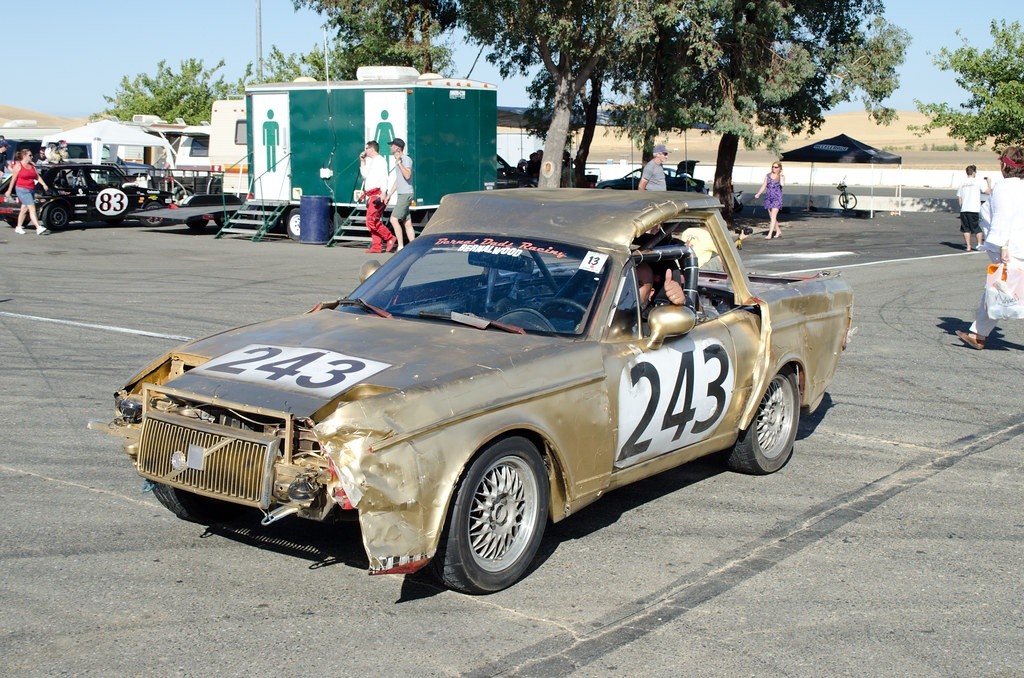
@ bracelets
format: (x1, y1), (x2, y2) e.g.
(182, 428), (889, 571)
(1000, 246), (1008, 249)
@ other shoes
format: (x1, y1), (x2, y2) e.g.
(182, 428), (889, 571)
(955, 330), (985, 350)
(365, 248), (382, 252)
(15, 226), (26, 234)
(36, 226), (47, 235)
(386, 236), (396, 252)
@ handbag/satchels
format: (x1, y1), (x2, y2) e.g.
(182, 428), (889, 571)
(984, 257), (1024, 319)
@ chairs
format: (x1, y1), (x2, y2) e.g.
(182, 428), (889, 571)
(648, 259), (687, 298)
(55, 171), (70, 187)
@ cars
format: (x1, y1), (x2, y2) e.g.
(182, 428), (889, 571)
(0, 140), (174, 233)
(111, 189), (847, 597)
(596, 160), (705, 194)
(495, 155), (527, 187)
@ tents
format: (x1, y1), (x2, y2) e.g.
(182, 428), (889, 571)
(779, 134), (902, 218)
(41, 119), (174, 169)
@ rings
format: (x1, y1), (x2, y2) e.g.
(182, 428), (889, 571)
(673, 295), (676, 299)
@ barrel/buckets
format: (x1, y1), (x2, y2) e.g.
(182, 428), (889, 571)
(299, 195), (330, 243)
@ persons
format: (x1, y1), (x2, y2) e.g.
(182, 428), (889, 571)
(955, 147), (1024, 350)
(32, 147), (49, 165)
(537, 150), (543, 161)
(5, 149), (48, 235)
(527, 153), (540, 188)
(635, 262), (693, 338)
(755, 161), (785, 239)
(956, 165), (991, 251)
(517, 159), (527, 173)
(384, 138), (415, 252)
(0, 140), (8, 181)
(562, 152), (576, 187)
(359, 141), (396, 253)
(638, 145), (672, 191)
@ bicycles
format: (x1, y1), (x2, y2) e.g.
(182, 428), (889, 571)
(837, 175), (857, 215)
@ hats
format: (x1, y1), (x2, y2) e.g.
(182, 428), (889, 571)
(653, 145), (672, 153)
(387, 138), (405, 149)
(0, 140), (11, 148)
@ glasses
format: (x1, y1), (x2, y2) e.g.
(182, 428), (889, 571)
(663, 152), (667, 156)
(29, 155), (32, 157)
(637, 280), (653, 288)
